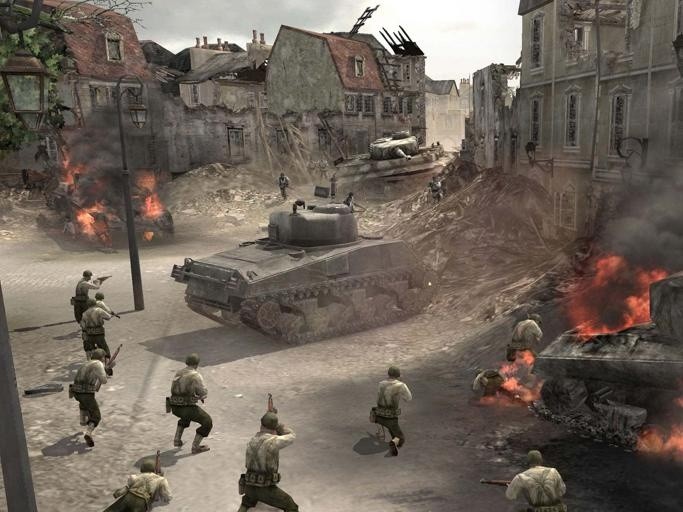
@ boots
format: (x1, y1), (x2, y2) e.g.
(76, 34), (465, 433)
(83, 421), (95, 447)
(173, 426), (183, 446)
(192, 433), (209, 454)
(389, 436), (400, 457)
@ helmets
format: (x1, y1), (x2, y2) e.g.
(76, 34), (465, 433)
(528, 314), (543, 324)
(260, 411), (279, 429)
(82, 270), (104, 307)
(184, 352), (200, 364)
(91, 348), (108, 360)
(527, 449), (543, 467)
(140, 459), (155, 472)
(387, 366), (401, 377)
